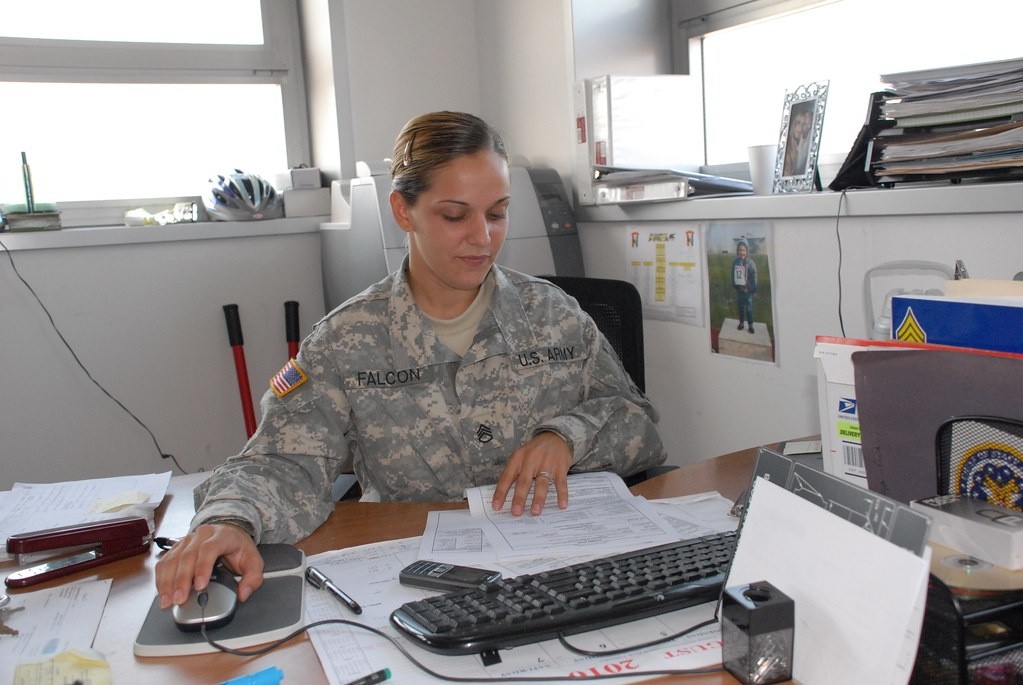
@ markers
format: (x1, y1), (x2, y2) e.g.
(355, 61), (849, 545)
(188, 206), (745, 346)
(341, 668), (392, 685)
(211, 666), (285, 685)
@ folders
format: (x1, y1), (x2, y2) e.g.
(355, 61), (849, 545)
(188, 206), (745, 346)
(572, 74), (755, 209)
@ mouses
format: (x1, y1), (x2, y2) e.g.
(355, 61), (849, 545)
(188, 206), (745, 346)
(171, 560), (240, 632)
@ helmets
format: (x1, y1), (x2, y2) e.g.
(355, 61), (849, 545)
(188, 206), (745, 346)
(737, 238), (749, 247)
(200, 169), (282, 222)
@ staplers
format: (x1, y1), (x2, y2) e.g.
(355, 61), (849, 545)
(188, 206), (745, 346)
(4, 517), (150, 591)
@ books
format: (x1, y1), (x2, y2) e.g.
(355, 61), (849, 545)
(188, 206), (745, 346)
(871, 57), (1023, 191)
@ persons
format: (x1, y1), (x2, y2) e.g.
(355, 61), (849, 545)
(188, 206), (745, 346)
(731, 237), (759, 333)
(783, 107), (815, 177)
(155, 111), (672, 611)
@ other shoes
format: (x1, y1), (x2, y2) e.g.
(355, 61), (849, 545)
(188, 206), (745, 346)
(738, 321), (744, 329)
(748, 322), (755, 333)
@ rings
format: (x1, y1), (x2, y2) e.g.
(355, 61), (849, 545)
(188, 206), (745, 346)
(536, 472), (554, 485)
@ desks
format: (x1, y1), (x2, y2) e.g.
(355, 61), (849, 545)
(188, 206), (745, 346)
(0, 433), (824, 685)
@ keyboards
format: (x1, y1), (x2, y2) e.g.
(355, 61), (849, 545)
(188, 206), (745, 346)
(389, 529), (737, 667)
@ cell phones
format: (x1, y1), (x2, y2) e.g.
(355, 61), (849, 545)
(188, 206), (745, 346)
(400, 561), (502, 592)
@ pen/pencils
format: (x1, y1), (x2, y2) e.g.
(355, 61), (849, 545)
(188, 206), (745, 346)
(305, 566), (363, 615)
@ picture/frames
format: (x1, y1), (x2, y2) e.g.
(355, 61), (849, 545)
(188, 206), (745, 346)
(772, 80), (829, 193)
